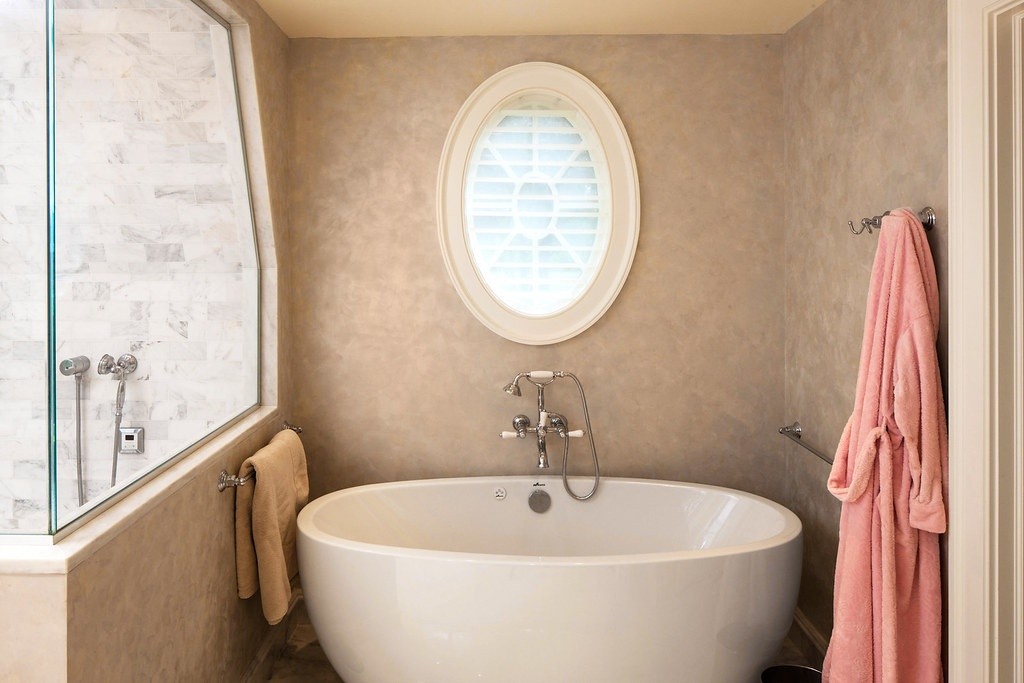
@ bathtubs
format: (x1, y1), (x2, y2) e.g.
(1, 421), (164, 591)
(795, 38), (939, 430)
(294, 479), (803, 683)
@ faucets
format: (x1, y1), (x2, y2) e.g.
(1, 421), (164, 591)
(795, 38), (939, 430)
(531, 412), (554, 468)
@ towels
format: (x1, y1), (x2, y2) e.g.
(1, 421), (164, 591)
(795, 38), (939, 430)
(236, 430), (314, 625)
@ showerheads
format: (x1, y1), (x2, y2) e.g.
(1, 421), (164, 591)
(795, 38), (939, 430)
(504, 369), (562, 397)
(97, 354), (126, 420)
